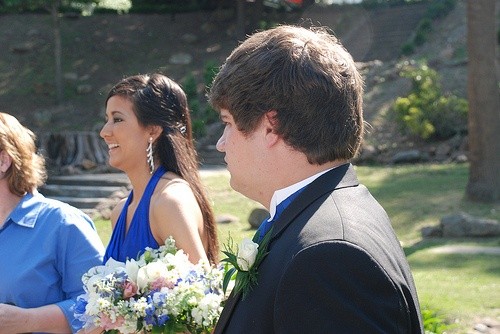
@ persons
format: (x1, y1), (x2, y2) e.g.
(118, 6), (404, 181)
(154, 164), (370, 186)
(208, 25), (424, 334)
(102, 72), (222, 326)
(0, 112), (107, 334)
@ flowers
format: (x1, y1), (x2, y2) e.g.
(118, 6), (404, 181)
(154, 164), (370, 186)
(219, 227), (273, 303)
(69, 235), (236, 334)
